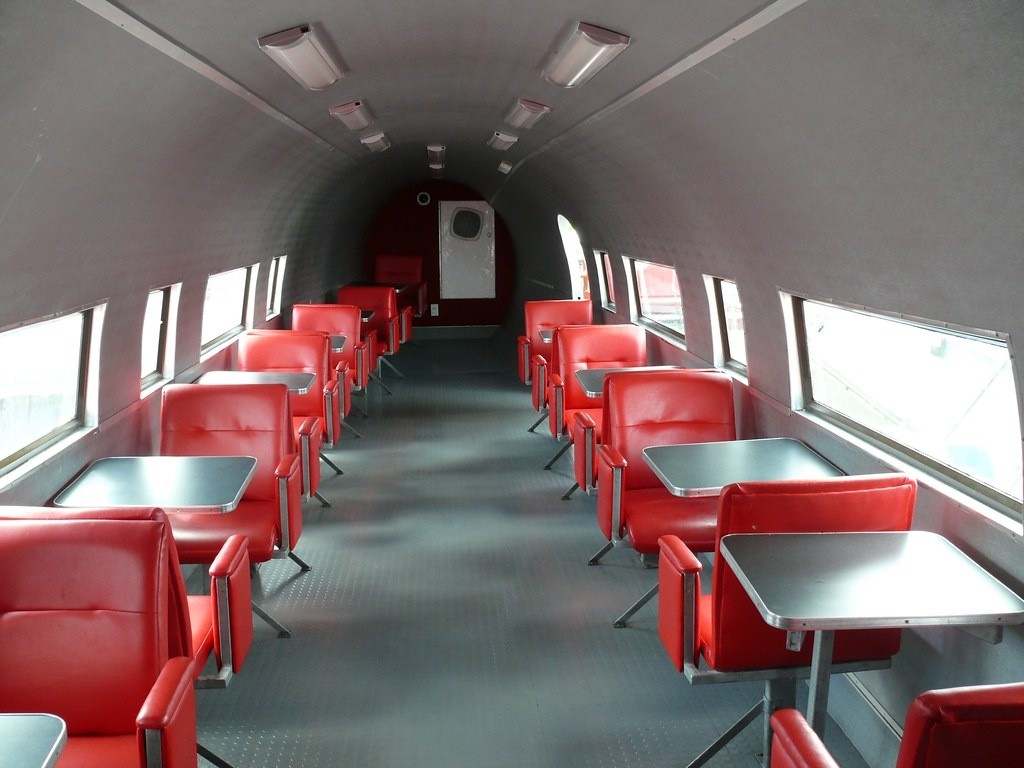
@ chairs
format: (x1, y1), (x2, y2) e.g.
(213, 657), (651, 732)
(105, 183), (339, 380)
(0, 254), (428, 768)
(769, 682), (1024, 768)
(517, 298), (735, 630)
(658, 473), (918, 768)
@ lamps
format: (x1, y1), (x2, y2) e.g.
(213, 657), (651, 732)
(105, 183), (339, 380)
(257, 23), (345, 95)
(540, 22), (630, 91)
(360, 133), (391, 153)
(426, 145), (446, 169)
(329, 99), (374, 134)
(504, 99), (550, 130)
(486, 132), (518, 152)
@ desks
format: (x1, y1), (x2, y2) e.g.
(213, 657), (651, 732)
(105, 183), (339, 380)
(191, 371), (317, 395)
(642, 436), (847, 498)
(0, 713), (68, 768)
(538, 329), (563, 344)
(575, 366), (686, 399)
(51, 456), (258, 515)
(719, 530), (1024, 744)
(330, 335), (348, 353)
(361, 311), (375, 322)
